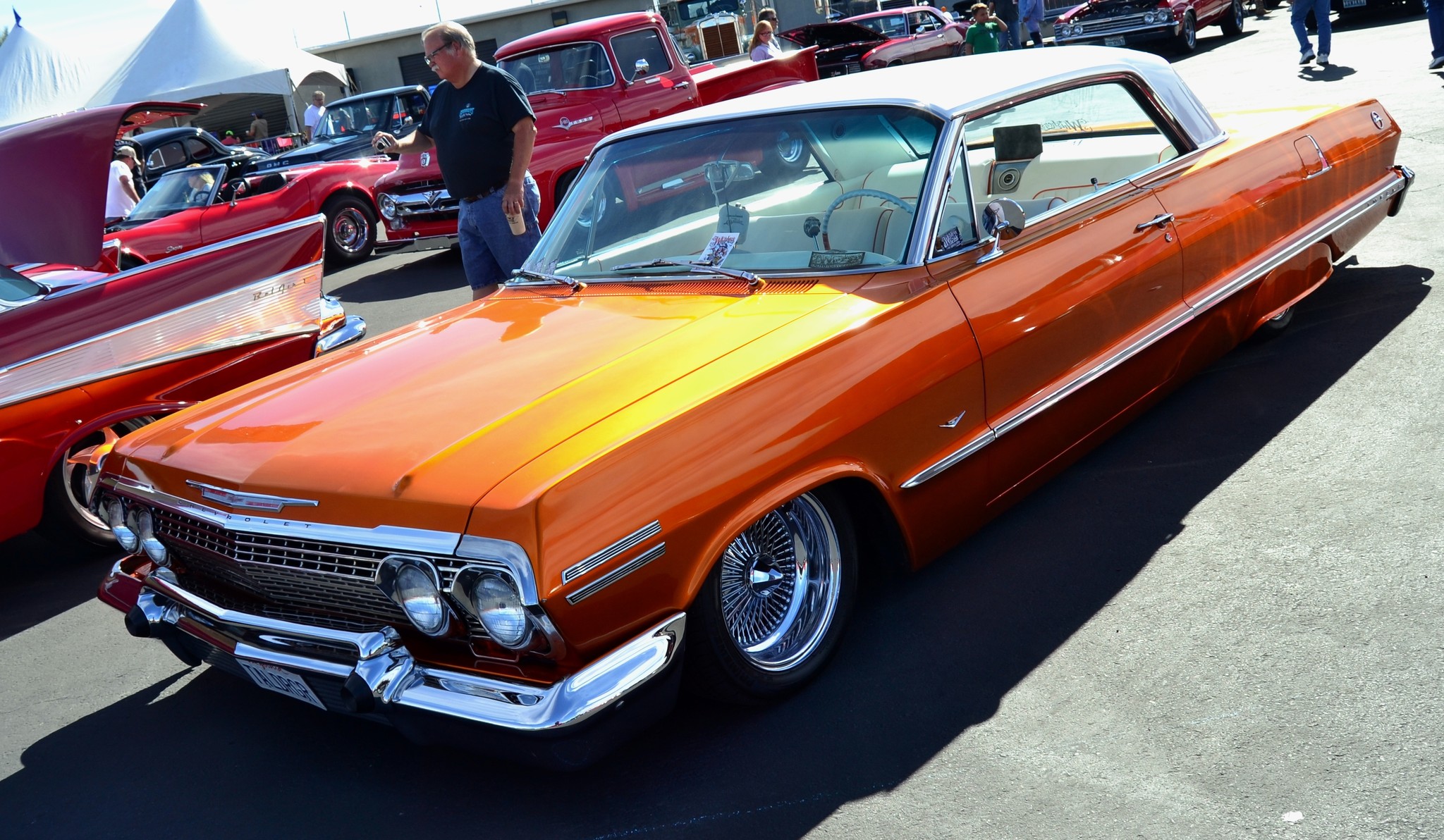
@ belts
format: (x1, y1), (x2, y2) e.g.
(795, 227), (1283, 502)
(464, 177), (509, 204)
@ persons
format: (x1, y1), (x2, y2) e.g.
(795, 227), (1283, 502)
(1422, 0), (1444, 69)
(748, 20), (783, 62)
(509, 59), (536, 94)
(989, 0), (1044, 51)
(304, 90), (335, 143)
(965, 3), (1008, 55)
(113, 136), (147, 200)
(335, 109), (354, 131)
(105, 146), (141, 223)
(562, 48), (579, 84)
(185, 164), (214, 202)
(371, 21), (542, 302)
(221, 131), (239, 145)
(247, 109), (268, 147)
(758, 8), (782, 52)
(1287, 0), (1331, 64)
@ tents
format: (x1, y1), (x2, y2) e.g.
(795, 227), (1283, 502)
(0, 7), (109, 130)
(83, 0), (354, 124)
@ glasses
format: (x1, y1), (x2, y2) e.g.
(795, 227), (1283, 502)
(766, 15), (777, 21)
(315, 98), (324, 102)
(425, 41), (452, 65)
(759, 31), (772, 36)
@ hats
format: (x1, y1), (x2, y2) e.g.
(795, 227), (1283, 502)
(117, 146), (142, 166)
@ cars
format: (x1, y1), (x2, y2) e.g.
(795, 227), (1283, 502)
(91, 47), (1416, 733)
(1052, 0), (1252, 59)
(1, 86), (433, 539)
(774, 7), (975, 77)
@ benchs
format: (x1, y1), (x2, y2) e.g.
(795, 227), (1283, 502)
(851, 144), (1178, 203)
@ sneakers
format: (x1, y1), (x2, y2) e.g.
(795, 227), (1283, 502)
(1319, 53), (1328, 64)
(1300, 49), (1315, 65)
(1430, 56), (1444, 70)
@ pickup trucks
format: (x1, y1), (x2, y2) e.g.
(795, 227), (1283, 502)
(370, 12), (821, 241)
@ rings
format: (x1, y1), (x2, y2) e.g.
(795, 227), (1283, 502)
(514, 201), (519, 203)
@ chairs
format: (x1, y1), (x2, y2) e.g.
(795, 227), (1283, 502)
(255, 173), (286, 193)
(222, 175), (252, 202)
(881, 196), (1069, 262)
(716, 205), (892, 260)
